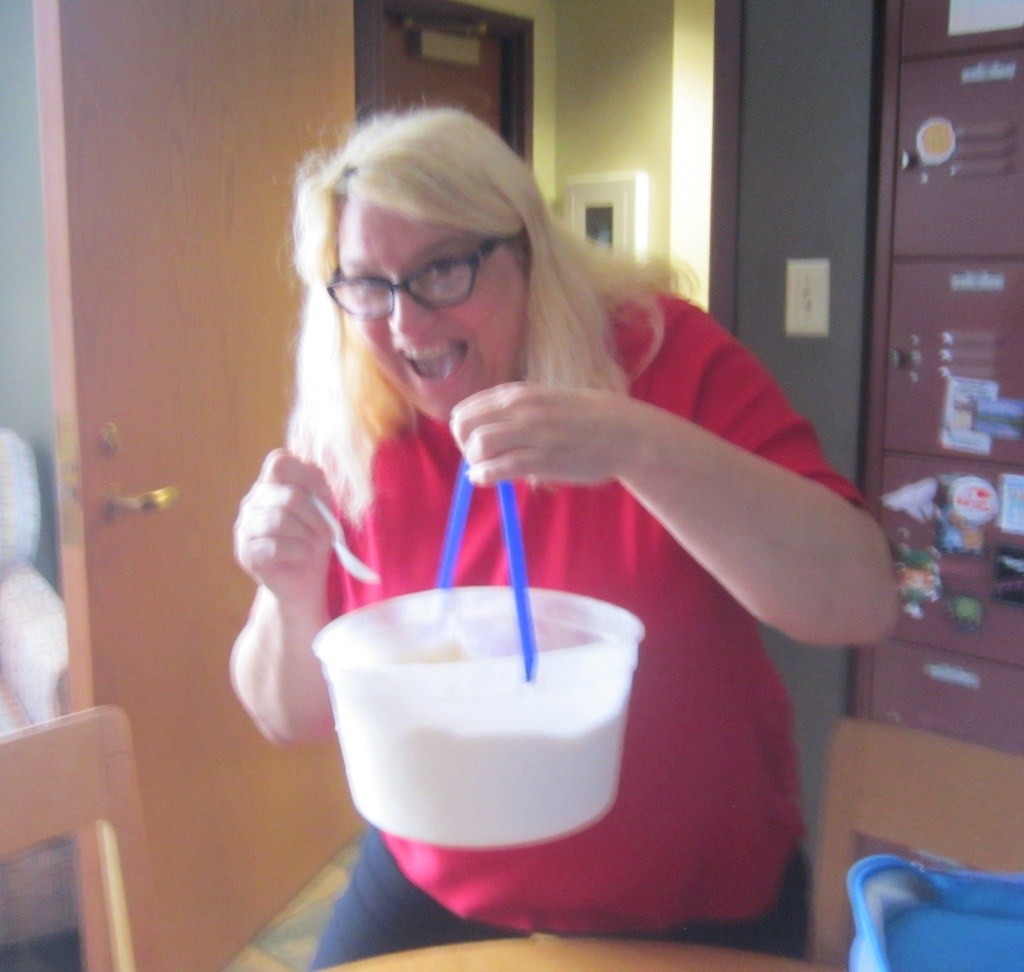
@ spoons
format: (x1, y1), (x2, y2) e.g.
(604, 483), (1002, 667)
(313, 496), (382, 588)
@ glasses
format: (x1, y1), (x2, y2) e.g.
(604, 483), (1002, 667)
(326, 235), (502, 323)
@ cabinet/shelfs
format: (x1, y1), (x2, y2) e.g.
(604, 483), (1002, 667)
(852, 0), (1023, 756)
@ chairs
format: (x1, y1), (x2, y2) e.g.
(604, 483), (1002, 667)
(810, 716), (1023, 969)
(0, 700), (168, 972)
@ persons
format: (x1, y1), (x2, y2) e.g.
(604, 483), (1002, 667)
(227, 106), (895, 971)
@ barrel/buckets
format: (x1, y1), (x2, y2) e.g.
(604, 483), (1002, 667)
(312, 436), (648, 852)
